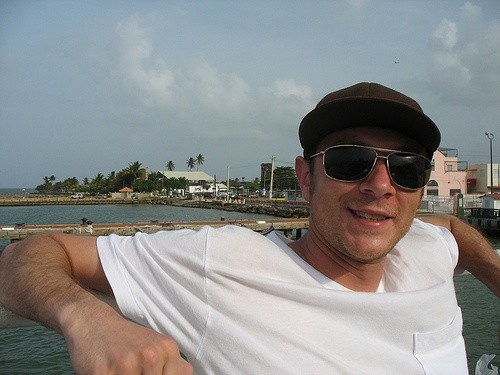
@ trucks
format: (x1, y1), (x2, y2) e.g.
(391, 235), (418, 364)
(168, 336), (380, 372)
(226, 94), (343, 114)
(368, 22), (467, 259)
(71, 193), (83, 199)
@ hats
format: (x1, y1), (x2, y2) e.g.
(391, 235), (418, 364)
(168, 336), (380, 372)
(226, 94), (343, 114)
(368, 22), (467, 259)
(298, 82), (441, 153)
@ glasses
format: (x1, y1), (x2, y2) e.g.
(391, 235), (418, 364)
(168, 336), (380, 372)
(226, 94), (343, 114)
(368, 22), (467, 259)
(310, 144), (436, 191)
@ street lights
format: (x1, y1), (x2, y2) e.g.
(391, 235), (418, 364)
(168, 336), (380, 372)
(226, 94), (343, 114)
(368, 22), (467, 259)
(485, 132), (495, 194)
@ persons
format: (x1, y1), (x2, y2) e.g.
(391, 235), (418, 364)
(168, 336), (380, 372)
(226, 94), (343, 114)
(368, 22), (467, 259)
(0, 82), (500, 375)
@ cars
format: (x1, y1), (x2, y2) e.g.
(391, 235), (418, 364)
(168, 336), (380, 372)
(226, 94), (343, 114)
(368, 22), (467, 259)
(94, 194), (107, 199)
